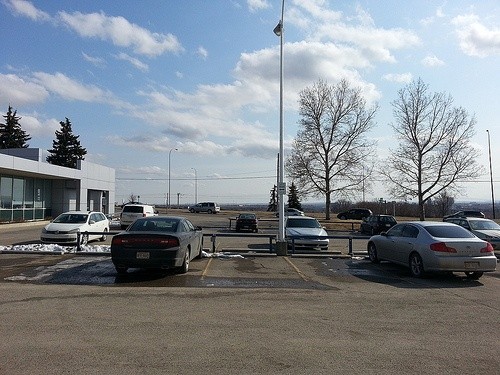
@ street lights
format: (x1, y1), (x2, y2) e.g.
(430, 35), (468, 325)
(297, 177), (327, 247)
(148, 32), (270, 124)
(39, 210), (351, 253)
(169, 148), (179, 209)
(486, 130), (495, 219)
(192, 167), (197, 205)
(272, 19), (288, 256)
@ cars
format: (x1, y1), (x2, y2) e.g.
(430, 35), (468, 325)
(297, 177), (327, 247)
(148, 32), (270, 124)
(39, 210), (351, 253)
(111, 216), (204, 274)
(359, 214), (398, 236)
(446, 216), (500, 256)
(274, 208), (305, 218)
(366, 220), (497, 280)
(284, 215), (330, 254)
(40, 210), (110, 247)
(236, 212), (260, 233)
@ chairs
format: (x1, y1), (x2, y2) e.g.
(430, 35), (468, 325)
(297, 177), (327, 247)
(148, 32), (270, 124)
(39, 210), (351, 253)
(146, 222), (156, 227)
(241, 215), (245, 218)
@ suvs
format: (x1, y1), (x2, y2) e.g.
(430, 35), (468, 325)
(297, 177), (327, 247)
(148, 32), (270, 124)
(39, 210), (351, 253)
(443, 210), (485, 223)
(337, 208), (373, 220)
(188, 202), (220, 214)
(119, 203), (160, 230)
